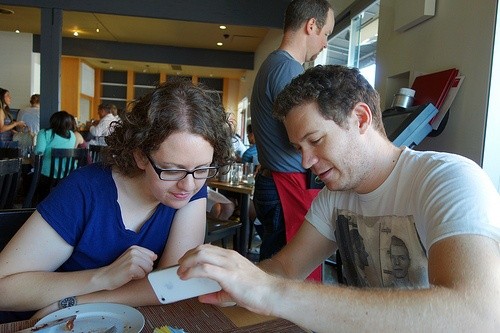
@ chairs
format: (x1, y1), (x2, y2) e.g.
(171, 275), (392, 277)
(205, 209), (242, 250)
(23, 150), (45, 209)
(47, 145), (88, 193)
(0, 158), (23, 210)
(88, 144), (112, 164)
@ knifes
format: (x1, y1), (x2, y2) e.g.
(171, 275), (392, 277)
(16, 315), (76, 333)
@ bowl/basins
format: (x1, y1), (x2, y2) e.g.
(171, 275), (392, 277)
(390, 94), (416, 108)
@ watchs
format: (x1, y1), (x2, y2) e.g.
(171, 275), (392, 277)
(58, 297), (77, 309)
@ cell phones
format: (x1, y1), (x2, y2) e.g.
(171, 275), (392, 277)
(146, 263), (223, 305)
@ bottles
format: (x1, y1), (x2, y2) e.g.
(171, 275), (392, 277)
(230, 162), (238, 186)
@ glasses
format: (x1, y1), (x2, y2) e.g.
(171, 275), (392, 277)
(142, 146), (219, 181)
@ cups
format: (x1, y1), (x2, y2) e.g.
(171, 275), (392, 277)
(237, 163), (244, 184)
(245, 162), (253, 179)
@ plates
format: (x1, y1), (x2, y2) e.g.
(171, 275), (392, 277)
(242, 180), (248, 184)
(34, 302), (145, 333)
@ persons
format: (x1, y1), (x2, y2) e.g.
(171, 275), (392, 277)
(250, 0), (337, 283)
(32, 110), (93, 191)
(16, 94), (40, 137)
(180, 65), (500, 333)
(83, 103), (123, 163)
(0, 88), (27, 158)
(205, 122), (256, 223)
(0, 77), (234, 320)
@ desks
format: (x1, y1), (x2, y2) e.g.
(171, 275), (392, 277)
(0, 294), (317, 333)
(206, 176), (256, 257)
